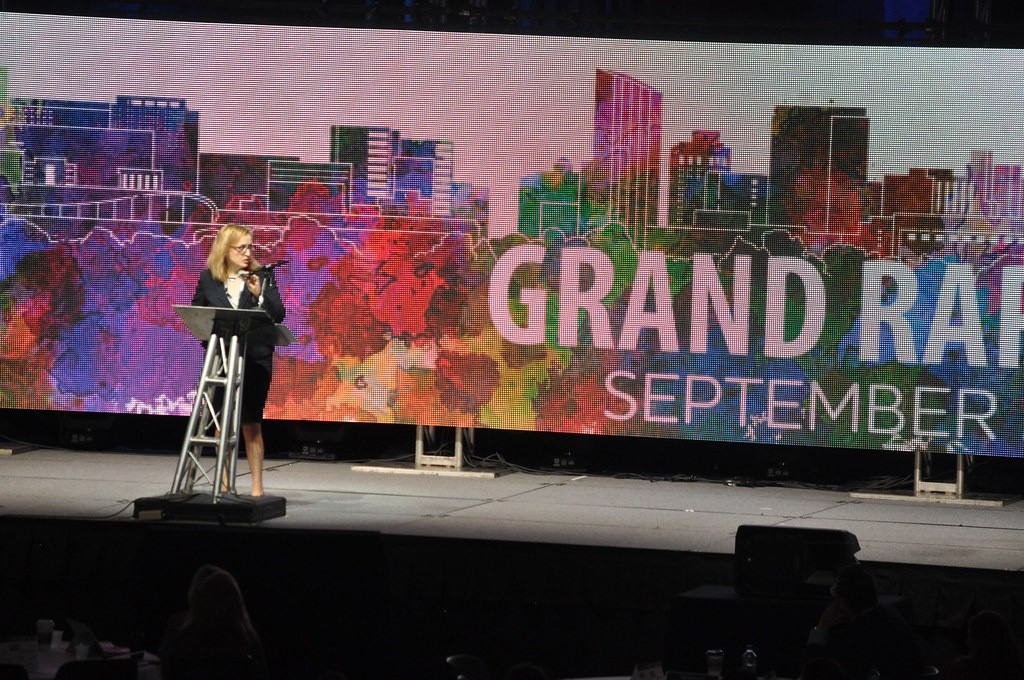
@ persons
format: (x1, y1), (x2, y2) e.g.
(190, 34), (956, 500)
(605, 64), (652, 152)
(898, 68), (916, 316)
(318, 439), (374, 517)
(191, 224), (287, 498)
(157, 562), (1022, 680)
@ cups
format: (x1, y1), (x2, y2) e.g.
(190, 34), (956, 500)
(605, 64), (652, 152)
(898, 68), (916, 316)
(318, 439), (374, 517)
(36, 619), (55, 652)
(705, 650), (724, 678)
(75, 644), (89, 660)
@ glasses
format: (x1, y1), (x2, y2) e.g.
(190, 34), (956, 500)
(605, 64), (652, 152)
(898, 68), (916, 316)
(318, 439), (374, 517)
(229, 244), (253, 252)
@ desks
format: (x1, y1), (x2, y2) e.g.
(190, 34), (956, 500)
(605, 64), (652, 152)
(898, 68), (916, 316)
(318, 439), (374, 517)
(683, 585), (912, 657)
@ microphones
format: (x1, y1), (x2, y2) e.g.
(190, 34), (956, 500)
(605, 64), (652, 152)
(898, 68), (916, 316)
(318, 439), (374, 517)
(238, 270), (248, 283)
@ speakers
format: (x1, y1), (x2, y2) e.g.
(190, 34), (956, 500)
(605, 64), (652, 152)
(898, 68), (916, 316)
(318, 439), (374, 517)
(734, 525), (862, 575)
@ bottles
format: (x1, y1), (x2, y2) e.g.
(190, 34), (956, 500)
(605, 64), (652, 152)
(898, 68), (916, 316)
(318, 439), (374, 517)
(742, 645), (757, 674)
